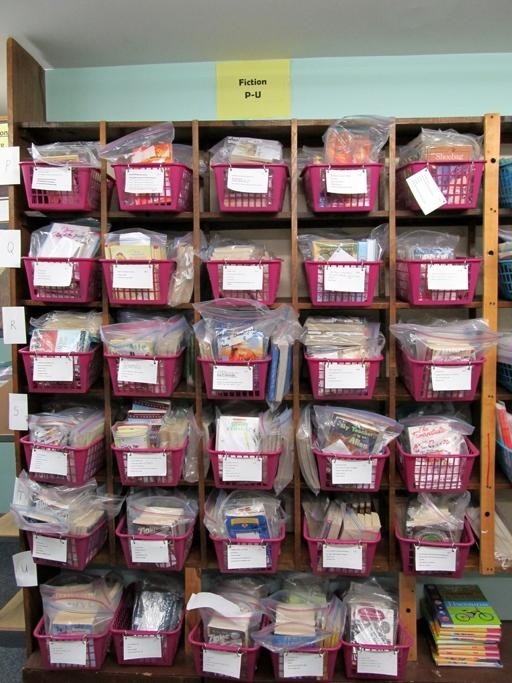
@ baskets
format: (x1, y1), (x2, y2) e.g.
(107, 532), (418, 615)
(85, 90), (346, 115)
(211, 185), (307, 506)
(104, 344), (188, 399)
(117, 514), (196, 573)
(498, 155), (512, 210)
(394, 256), (484, 307)
(310, 435), (391, 492)
(18, 342), (102, 395)
(395, 437), (480, 495)
(33, 614), (109, 670)
(208, 522), (285, 576)
(210, 161), (291, 215)
(200, 258), (285, 307)
(111, 437), (190, 488)
(498, 257), (512, 302)
(395, 159), (490, 211)
(23, 520), (109, 572)
(301, 260), (386, 308)
(497, 361), (512, 394)
(109, 595), (182, 667)
(343, 623), (414, 682)
(188, 618), (266, 682)
(207, 436), (281, 491)
(109, 160), (207, 214)
(18, 433), (512, 683)
(196, 352), (273, 402)
(393, 518), (476, 579)
(302, 518), (382, 577)
(18, 428), (105, 487)
(298, 163), (384, 215)
(98, 258), (177, 307)
(19, 158), (116, 214)
(304, 347), (385, 401)
(273, 620), (342, 683)
(396, 343), (487, 402)
(21, 255), (102, 303)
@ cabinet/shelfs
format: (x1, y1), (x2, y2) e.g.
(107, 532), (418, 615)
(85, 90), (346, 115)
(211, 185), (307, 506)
(0, 115), (512, 682)
